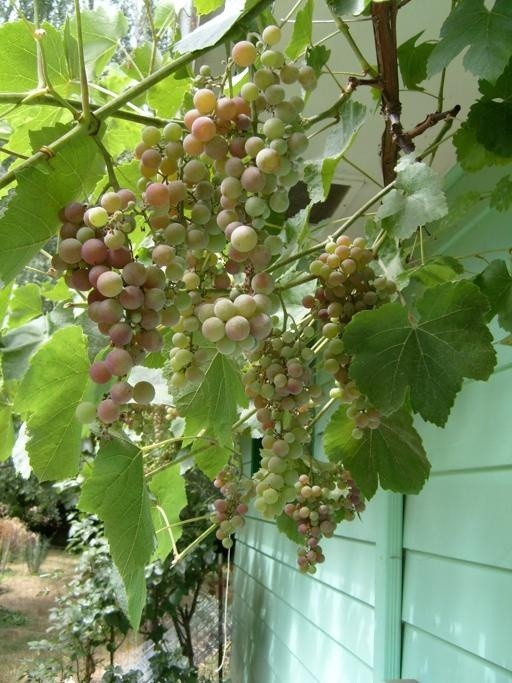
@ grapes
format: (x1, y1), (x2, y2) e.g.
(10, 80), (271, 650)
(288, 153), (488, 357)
(50, 23), (396, 575)
